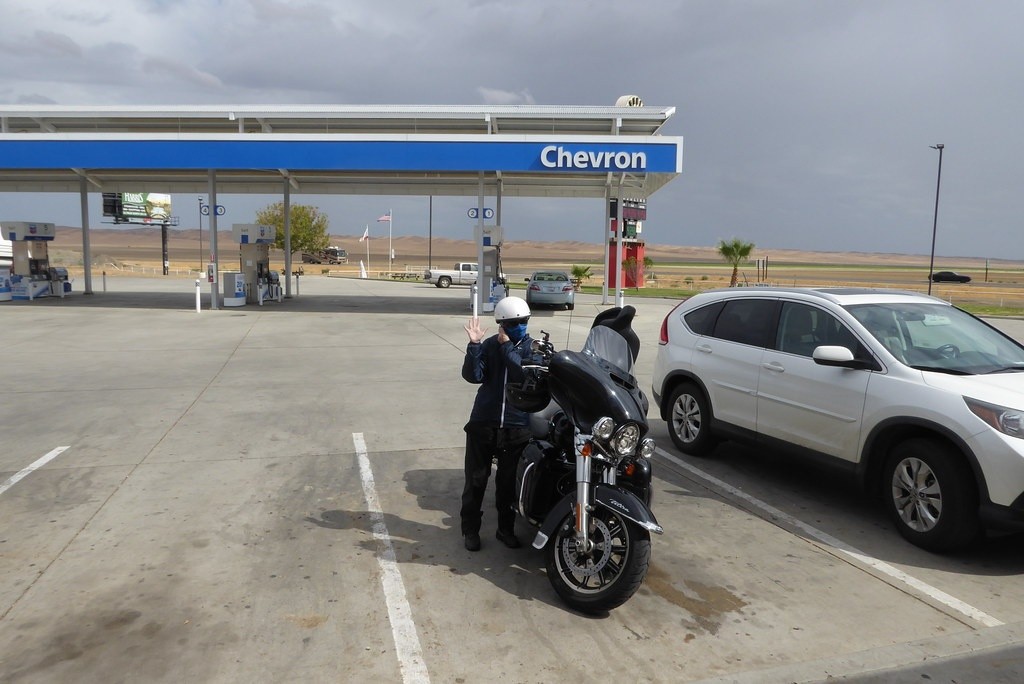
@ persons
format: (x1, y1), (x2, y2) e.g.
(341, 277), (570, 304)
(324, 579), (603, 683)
(460, 296), (538, 551)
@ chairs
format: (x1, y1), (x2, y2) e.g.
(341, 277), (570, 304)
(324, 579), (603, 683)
(784, 308), (820, 358)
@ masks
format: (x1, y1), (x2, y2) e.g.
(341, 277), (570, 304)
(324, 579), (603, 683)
(504, 324), (527, 341)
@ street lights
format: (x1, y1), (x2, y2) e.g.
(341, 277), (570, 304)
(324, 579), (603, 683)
(928, 143), (945, 295)
(197, 196), (203, 272)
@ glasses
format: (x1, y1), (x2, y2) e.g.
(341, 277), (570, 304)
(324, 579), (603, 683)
(502, 317), (527, 327)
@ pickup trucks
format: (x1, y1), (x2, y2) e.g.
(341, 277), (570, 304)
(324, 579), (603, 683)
(424, 262), (506, 288)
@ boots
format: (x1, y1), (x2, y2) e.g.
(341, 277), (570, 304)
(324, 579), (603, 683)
(496, 509), (522, 548)
(461, 511), (484, 551)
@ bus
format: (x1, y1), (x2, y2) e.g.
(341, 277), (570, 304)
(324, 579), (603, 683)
(302, 246), (346, 265)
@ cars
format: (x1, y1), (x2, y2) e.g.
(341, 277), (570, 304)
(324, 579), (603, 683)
(928, 272), (971, 283)
(524, 270), (576, 311)
(649, 288), (1023, 559)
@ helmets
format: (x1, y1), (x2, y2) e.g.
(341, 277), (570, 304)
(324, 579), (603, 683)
(494, 296), (531, 324)
(505, 382), (551, 413)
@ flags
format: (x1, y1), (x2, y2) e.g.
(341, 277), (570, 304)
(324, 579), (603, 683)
(359, 228), (368, 242)
(377, 211), (390, 222)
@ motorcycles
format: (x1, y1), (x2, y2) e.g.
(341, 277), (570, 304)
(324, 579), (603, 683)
(508, 327), (665, 618)
